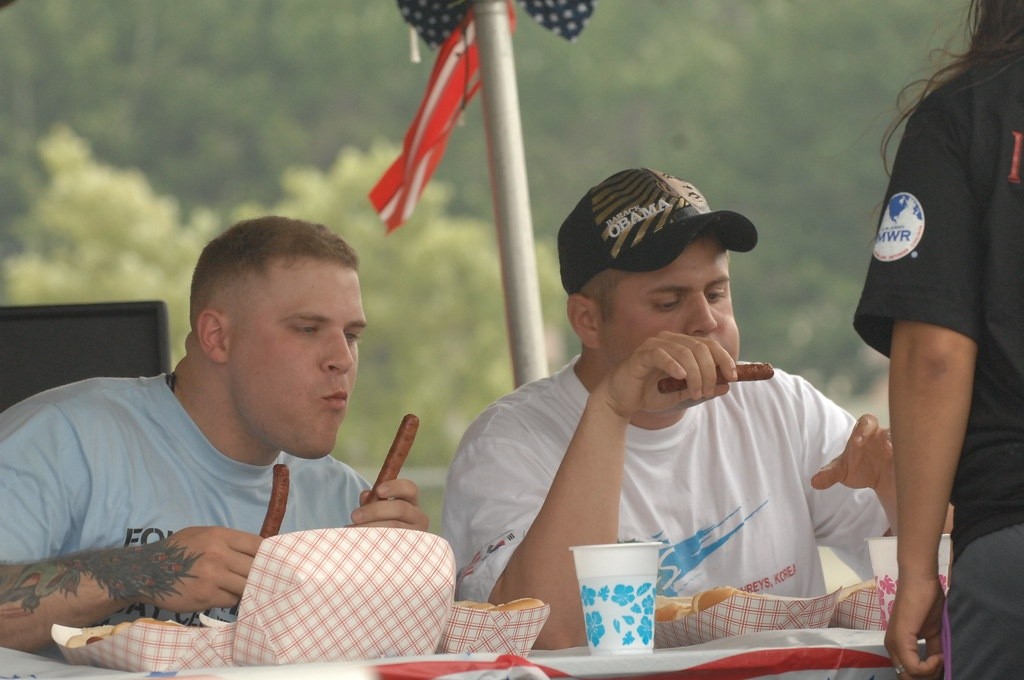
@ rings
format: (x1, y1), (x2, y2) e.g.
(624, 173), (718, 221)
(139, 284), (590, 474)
(894, 664), (906, 675)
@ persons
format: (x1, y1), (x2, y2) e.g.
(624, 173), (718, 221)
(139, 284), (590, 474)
(439, 168), (957, 652)
(849, 0), (1024, 680)
(0, 213), (436, 664)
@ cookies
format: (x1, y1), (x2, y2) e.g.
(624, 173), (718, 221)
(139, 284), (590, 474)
(658, 363), (774, 394)
(259, 464), (289, 539)
(362, 413), (419, 503)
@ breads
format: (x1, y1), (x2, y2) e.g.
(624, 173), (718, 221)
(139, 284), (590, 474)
(654, 586), (767, 622)
(452, 597), (545, 612)
(835, 578), (876, 602)
(64, 617), (182, 647)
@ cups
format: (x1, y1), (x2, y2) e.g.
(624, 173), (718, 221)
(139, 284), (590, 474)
(568, 541), (664, 654)
(864, 533), (950, 631)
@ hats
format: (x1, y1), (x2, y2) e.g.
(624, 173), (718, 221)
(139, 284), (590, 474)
(557, 167), (759, 294)
